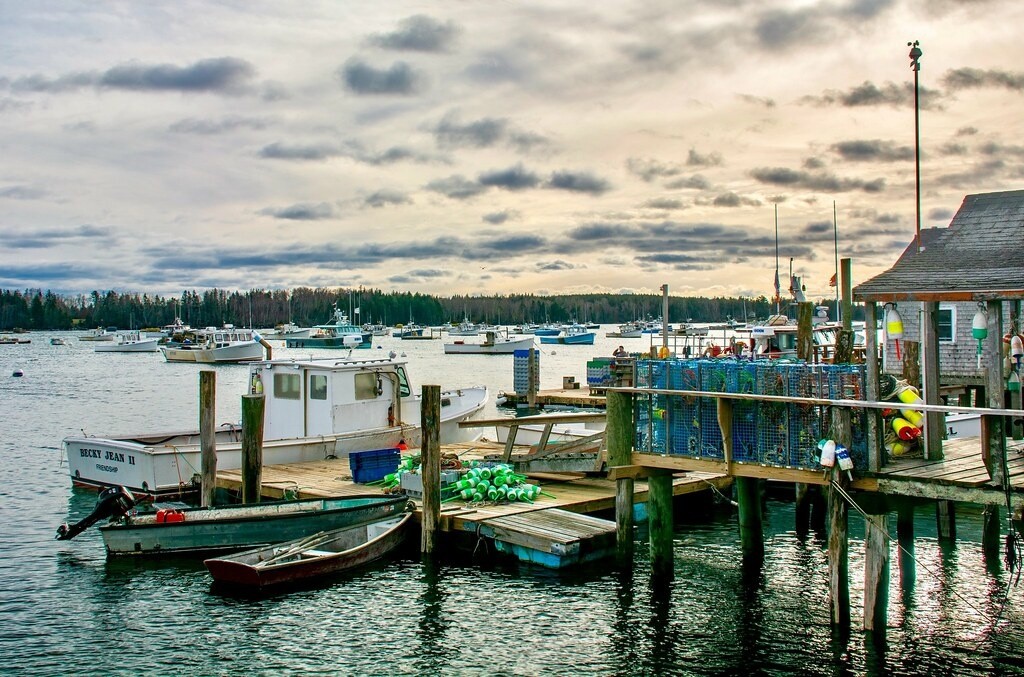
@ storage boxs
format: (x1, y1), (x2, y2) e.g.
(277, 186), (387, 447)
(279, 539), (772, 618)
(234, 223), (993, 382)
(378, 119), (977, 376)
(348, 447), (401, 483)
(633, 359), (869, 471)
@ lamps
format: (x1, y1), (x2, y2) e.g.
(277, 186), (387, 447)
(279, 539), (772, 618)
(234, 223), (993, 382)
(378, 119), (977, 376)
(265, 364), (272, 369)
(389, 351), (397, 359)
(294, 365), (300, 369)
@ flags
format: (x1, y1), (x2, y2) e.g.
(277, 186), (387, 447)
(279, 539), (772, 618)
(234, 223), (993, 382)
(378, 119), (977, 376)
(332, 300), (336, 305)
(355, 308), (359, 313)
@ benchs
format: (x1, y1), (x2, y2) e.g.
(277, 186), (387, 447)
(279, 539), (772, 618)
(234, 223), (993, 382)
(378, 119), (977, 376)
(281, 547), (336, 557)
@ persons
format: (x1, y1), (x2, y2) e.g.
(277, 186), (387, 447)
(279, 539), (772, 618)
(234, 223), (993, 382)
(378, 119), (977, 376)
(613, 346), (626, 356)
(763, 338), (782, 353)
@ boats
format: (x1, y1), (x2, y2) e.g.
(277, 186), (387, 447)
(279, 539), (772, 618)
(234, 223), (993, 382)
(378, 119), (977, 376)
(441, 317), (601, 355)
(0, 327), (31, 344)
(749, 305), (866, 359)
(98, 494), (409, 556)
(159, 300), (390, 365)
(605, 315), (709, 338)
(95, 331), (158, 352)
(78, 326), (113, 342)
(392, 321), (423, 338)
(203, 509), (413, 602)
(62, 330), (488, 502)
(708, 316), (746, 330)
